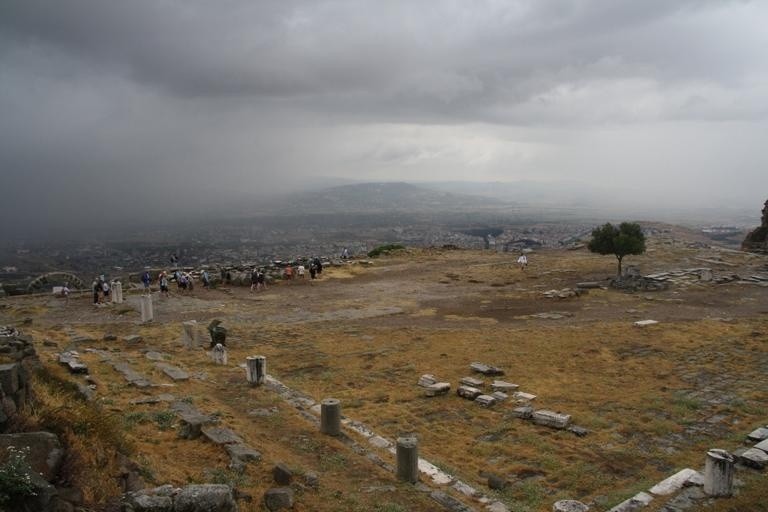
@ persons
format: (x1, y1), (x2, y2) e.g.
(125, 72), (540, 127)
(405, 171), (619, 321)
(63, 285), (71, 305)
(517, 252), (527, 271)
(91, 257), (323, 306)
